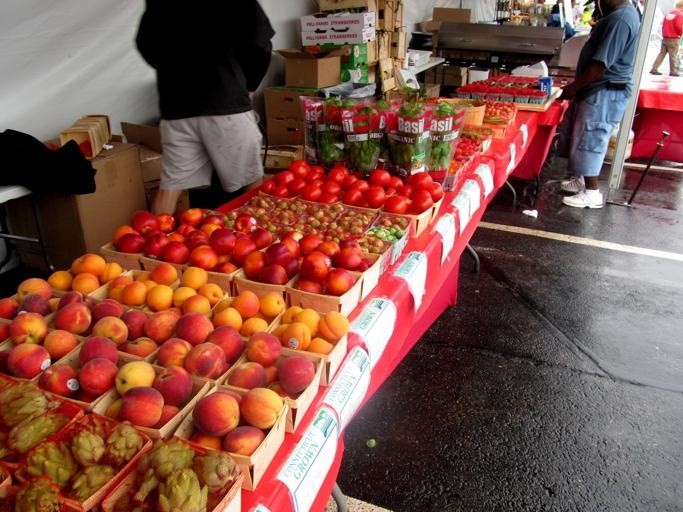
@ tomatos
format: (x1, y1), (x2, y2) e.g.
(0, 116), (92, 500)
(447, 132), (481, 176)
(260, 160), (444, 214)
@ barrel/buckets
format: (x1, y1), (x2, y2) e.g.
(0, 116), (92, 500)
(409, 31), (434, 84)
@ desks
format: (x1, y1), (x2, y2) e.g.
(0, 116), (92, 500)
(621, 74), (683, 175)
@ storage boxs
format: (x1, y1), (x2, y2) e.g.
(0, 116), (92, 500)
(215, 84), (518, 275)
(402, 6), (475, 88)
(257, 0), (412, 83)
(42, 114), (191, 271)
(2, 239), (382, 511)
(459, 85), (551, 106)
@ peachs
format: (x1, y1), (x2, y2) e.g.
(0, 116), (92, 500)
(0, 209), (372, 452)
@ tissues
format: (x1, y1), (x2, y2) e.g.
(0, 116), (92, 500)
(511, 60), (549, 77)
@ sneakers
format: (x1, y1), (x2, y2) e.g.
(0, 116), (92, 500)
(648, 70), (663, 76)
(559, 174), (589, 193)
(669, 75), (680, 78)
(561, 190), (604, 209)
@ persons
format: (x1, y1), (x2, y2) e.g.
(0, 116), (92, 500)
(536, 0), (641, 209)
(135, 0), (276, 216)
(650, 0), (683, 77)
